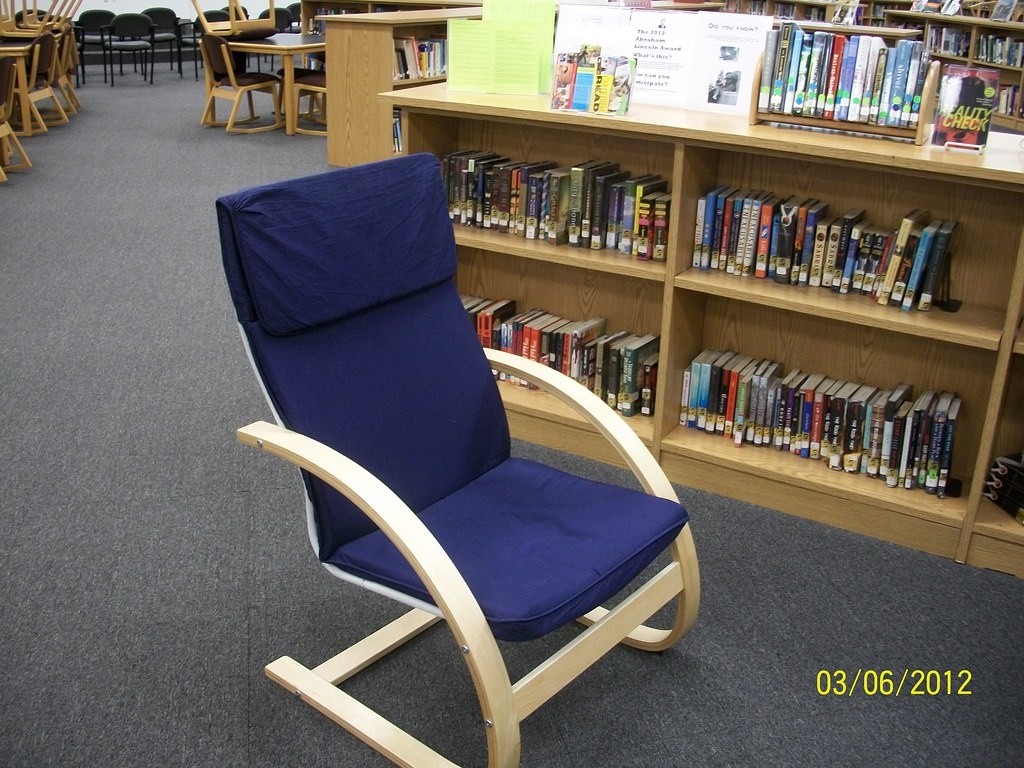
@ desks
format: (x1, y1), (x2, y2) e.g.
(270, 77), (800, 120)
(221, 33), (327, 135)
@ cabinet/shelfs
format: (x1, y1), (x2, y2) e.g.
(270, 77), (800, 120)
(377, 84), (1023, 584)
(721, 0), (1024, 124)
(315, 7), (485, 170)
(301, 0), (483, 74)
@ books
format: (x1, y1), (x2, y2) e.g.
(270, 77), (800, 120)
(679, 349), (963, 499)
(459, 294), (661, 417)
(756, 23), (928, 129)
(552, 47), (636, 115)
(393, 109), (402, 152)
(692, 185), (958, 312)
(722, 0), (1024, 67)
(441, 148), (670, 263)
(393, 33), (448, 81)
(307, 7), (384, 36)
(931, 62), (1024, 152)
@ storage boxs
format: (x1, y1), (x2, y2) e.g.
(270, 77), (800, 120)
(550, 44), (637, 116)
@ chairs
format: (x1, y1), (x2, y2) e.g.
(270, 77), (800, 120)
(0, 0), (327, 183)
(213, 150), (700, 767)
